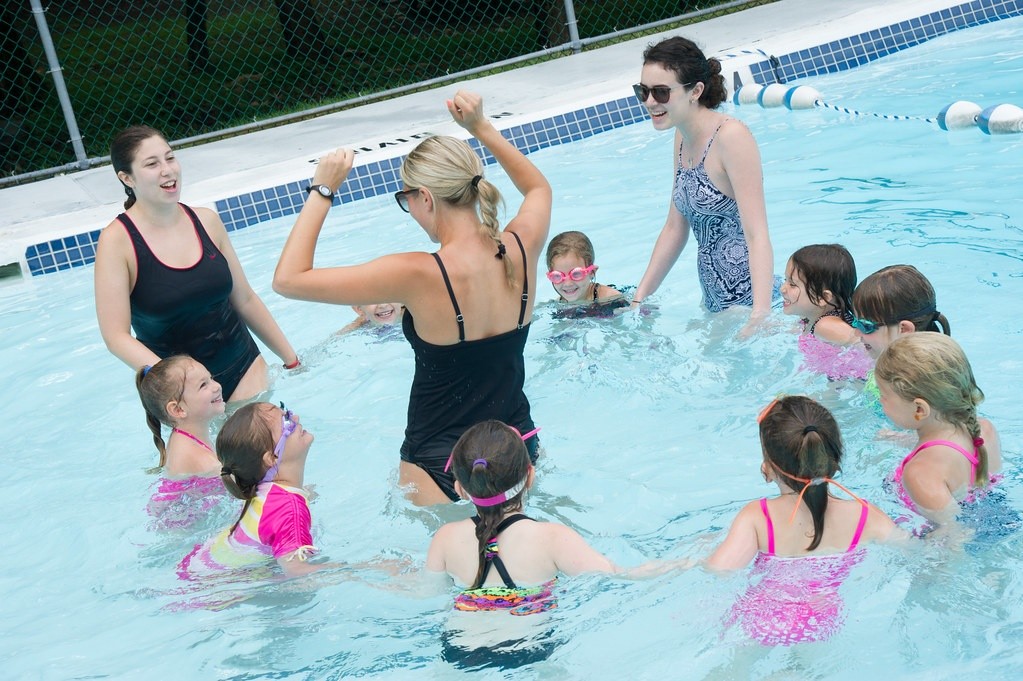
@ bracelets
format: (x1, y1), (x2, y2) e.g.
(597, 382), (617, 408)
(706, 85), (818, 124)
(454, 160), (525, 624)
(283, 356), (301, 370)
(632, 300), (641, 303)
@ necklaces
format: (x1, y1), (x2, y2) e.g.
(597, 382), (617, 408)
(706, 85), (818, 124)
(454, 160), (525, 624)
(683, 144), (693, 169)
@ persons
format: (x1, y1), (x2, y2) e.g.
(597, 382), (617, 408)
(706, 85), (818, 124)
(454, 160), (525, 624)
(873, 331), (1002, 511)
(846, 264), (951, 360)
(703, 394), (908, 571)
(94, 124), (302, 401)
(216, 401), (321, 575)
(545, 231), (621, 302)
(424, 418), (619, 590)
(135, 353), (226, 474)
(780, 244), (858, 345)
(352, 303), (404, 325)
(271, 90), (553, 508)
(631, 35), (784, 311)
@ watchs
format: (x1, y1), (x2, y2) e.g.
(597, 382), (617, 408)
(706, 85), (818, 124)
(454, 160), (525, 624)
(306, 184), (335, 203)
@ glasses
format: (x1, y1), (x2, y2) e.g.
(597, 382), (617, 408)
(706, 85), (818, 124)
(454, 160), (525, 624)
(281, 404), (296, 437)
(395, 187), (422, 212)
(632, 80), (697, 105)
(547, 265), (594, 284)
(757, 394), (780, 428)
(851, 315), (878, 334)
